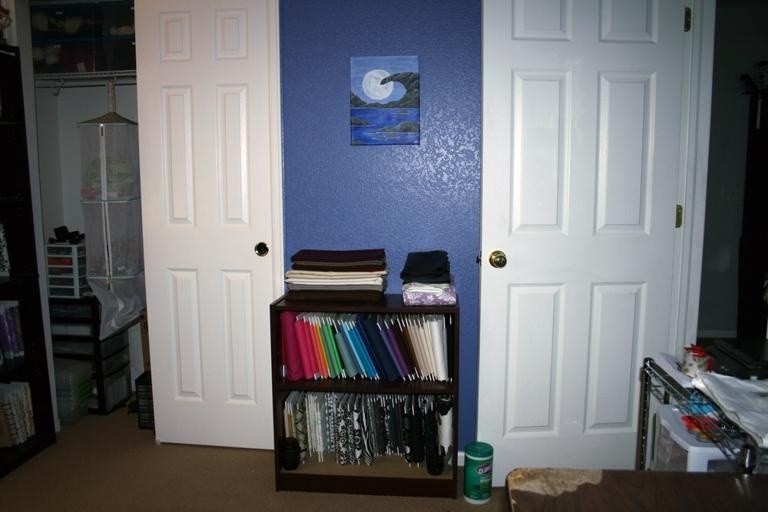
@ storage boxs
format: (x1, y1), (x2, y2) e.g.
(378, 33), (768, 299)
(649, 401), (740, 473)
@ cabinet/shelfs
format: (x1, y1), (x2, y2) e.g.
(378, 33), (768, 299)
(635, 358), (768, 472)
(45, 240), (90, 299)
(269, 294), (458, 498)
(49, 299), (131, 416)
(1, 43), (58, 482)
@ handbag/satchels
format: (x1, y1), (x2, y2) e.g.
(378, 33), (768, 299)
(703, 338), (762, 379)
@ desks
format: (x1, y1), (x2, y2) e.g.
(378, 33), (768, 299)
(504, 467), (768, 511)
